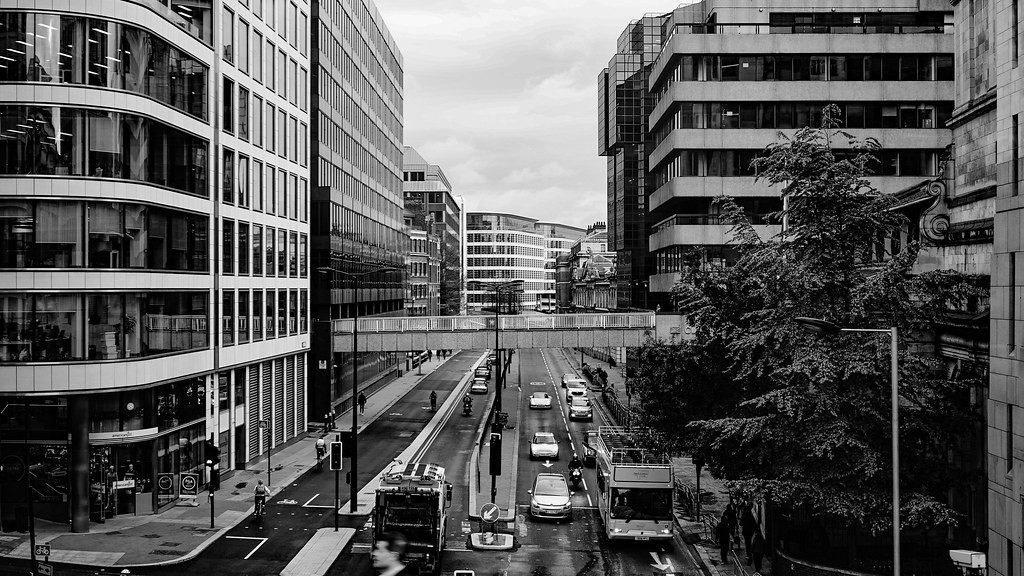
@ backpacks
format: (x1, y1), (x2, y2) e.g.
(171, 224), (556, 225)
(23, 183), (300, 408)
(317, 439), (324, 446)
(257, 485), (265, 494)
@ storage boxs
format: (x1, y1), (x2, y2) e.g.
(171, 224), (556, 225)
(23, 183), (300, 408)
(117, 350), (131, 358)
(102, 351), (118, 359)
(100, 331), (116, 339)
(100, 339), (116, 346)
(100, 345), (117, 352)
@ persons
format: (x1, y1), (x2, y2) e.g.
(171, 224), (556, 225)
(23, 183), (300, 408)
(437, 350), (452, 359)
(358, 391), (366, 416)
(253, 479), (271, 516)
(429, 391), (437, 409)
(428, 350), (432, 361)
(617, 496), (626, 506)
(486, 359), (492, 371)
(463, 393), (473, 412)
(568, 454), (583, 482)
(598, 368), (608, 387)
(373, 532), (418, 576)
(715, 501), (765, 572)
(315, 437), (327, 460)
(608, 356), (614, 369)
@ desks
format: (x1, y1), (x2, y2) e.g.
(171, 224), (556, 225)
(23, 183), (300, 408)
(0, 341), (33, 361)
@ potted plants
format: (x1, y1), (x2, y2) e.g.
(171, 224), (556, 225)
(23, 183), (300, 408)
(114, 312), (137, 350)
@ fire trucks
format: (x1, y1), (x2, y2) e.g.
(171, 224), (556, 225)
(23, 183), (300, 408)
(370, 462), (454, 576)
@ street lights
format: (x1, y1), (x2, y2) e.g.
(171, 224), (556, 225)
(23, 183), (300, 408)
(317, 266), (401, 513)
(795, 316), (900, 576)
(466, 280), (524, 426)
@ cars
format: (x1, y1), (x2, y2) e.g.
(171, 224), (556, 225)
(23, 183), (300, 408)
(474, 366), (491, 380)
(529, 391), (553, 410)
(485, 354), (497, 366)
(528, 431), (561, 462)
(470, 377), (490, 394)
(560, 373), (595, 422)
(527, 472), (576, 521)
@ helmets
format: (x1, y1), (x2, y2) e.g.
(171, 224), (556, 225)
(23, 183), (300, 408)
(466, 393), (470, 396)
(573, 455), (578, 461)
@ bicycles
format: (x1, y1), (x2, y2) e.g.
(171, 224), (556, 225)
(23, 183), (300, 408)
(316, 446), (328, 468)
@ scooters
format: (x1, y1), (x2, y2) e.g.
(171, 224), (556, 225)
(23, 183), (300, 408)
(486, 363), (493, 372)
(429, 397), (437, 412)
(464, 398), (474, 416)
(254, 494), (270, 523)
(568, 465), (584, 488)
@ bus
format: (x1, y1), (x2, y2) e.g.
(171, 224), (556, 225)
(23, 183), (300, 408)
(594, 426), (676, 542)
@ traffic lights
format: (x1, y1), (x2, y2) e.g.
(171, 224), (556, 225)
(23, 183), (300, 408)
(330, 442), (341, 470)
(204, 444), (215, 466)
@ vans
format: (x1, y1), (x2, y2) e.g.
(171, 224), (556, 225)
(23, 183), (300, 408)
(581, 430), (598, 467)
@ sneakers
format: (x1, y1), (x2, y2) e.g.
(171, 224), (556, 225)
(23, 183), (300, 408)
(359, 413), (362, 415)
(361, 413), (364, 416)
